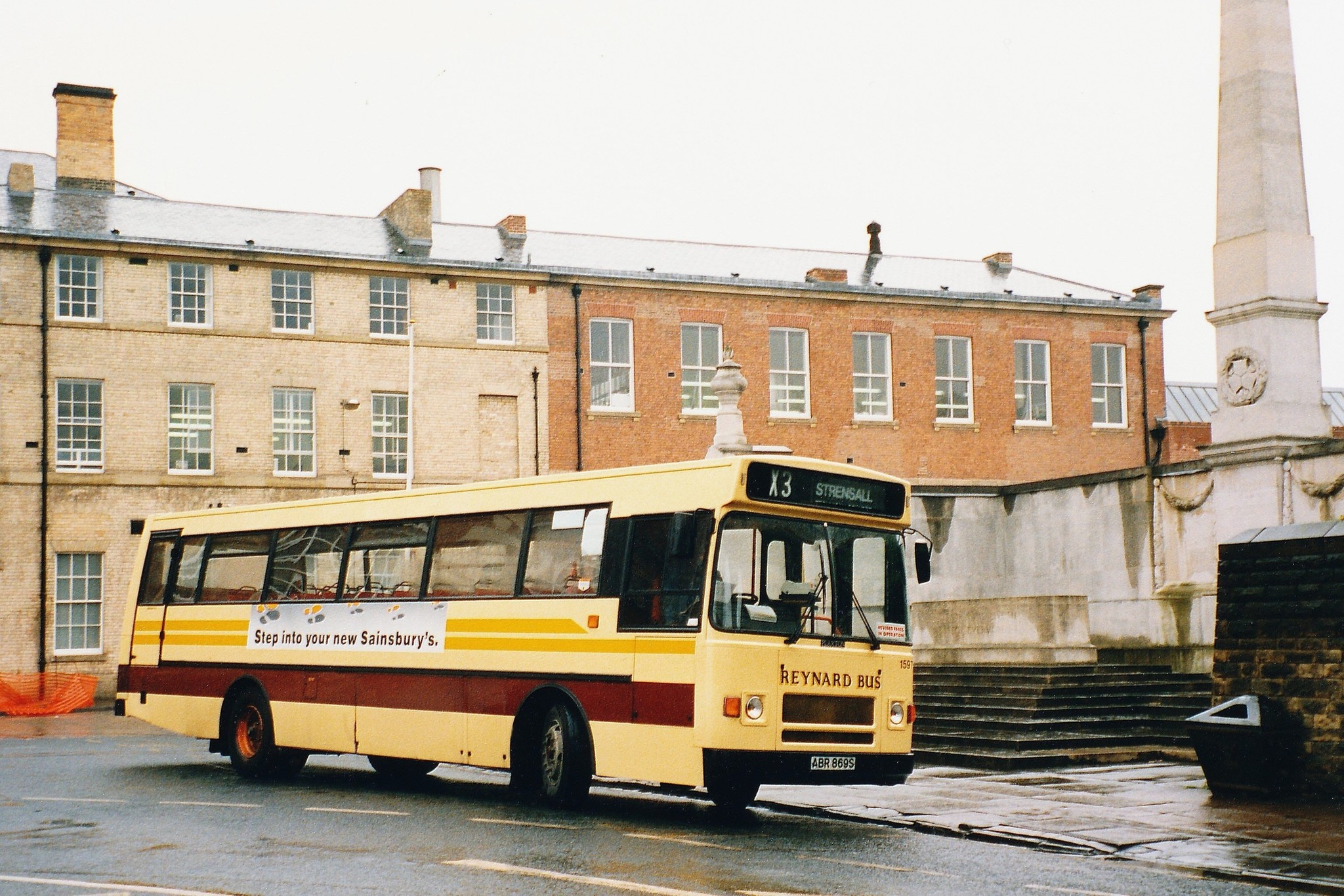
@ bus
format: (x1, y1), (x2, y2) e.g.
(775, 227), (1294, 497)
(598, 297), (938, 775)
(115, 450), (932, 808)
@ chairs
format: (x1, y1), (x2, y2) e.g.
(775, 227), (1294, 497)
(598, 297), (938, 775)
(186, 575), (594, 602)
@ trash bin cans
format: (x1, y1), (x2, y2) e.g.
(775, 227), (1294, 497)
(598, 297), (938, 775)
(1184, 696), (1270, 788)
